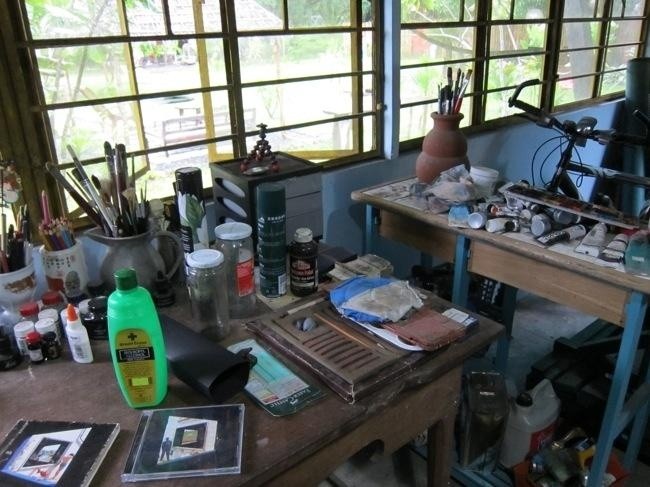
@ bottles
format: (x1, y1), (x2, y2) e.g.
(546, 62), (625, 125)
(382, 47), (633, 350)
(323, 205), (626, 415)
(529, 457), (560, 487)
(448, 166), (579, 237)
(621, 223), (650, 275)
(0, 167), (321, 410)
(417, 111), (470, 183)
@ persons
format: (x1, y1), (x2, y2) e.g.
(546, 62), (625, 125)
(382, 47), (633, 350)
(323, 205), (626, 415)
(51, 452), (73, 480)
(159, 437), (172, 461)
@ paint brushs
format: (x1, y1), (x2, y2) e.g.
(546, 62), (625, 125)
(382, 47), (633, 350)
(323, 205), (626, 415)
(45, 142), (150, 237)
(438, 67), (472, 115)
(279, 294), (328, 318)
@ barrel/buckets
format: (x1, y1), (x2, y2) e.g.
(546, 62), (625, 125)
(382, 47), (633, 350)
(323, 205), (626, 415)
(500, 379), (563, 468)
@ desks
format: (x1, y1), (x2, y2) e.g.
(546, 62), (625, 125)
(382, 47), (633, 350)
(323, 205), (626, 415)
(1, 242), (508, 486)
(352, 175), (649, 486)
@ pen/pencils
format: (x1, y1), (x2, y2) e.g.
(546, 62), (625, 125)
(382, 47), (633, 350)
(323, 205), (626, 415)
(312, 307), (389, 353)
(38, 188), (75, 251)
(0, 203), (29, 274)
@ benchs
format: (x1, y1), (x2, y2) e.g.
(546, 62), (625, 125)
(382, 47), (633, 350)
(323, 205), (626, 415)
(143, 107), (254, 157)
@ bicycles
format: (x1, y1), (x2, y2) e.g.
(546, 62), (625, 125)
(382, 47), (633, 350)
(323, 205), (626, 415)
(480, 72), (648, 376)
